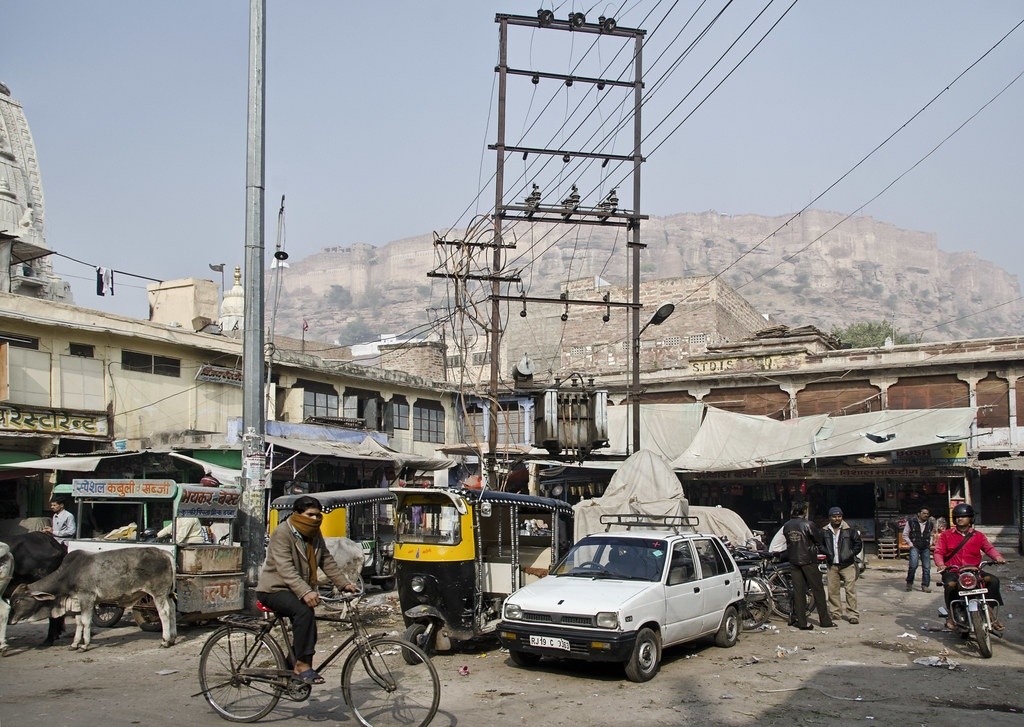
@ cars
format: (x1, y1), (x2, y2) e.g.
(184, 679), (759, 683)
(817, 550), (866, 584)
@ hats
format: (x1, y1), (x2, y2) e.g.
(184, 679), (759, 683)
(829, 507), (844, 516)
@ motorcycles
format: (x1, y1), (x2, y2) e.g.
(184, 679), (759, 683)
(268, 486), (397, 592)
(935, 559), (1006, 659)
(388, 479), (580, 666)
(141, 527), (173, 543)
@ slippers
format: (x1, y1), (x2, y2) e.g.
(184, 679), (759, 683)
(293, 669), (325, 685)
(988, 616), (1005, 631)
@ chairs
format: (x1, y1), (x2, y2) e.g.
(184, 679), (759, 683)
(654, 552), (687, 583)
(605, 550), (625, 576)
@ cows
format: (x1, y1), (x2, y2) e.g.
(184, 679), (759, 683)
(7, 547), (177, 653)
(246, 536), (365, 620)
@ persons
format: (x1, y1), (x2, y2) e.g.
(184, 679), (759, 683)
(42, 497), (77, 541)
(255, 495), (361, 684)
(901, 506), (934, 593)
(769, 525), (790, 563)
(933, 503), (1008, 631)
(783, 502), (838, 630)
(818, 507), (862, 624)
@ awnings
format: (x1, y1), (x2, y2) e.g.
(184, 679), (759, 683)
(238, 431), (458, 471)
(522, 402), (979, 473)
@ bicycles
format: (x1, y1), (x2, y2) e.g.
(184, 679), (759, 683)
(197, 591), (442, 727)
(720, 536), (815, 630)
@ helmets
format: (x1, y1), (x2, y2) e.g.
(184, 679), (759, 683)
(952, 503), (975, 525)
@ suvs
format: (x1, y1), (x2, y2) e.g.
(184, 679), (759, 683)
(495, 514), (744, 682)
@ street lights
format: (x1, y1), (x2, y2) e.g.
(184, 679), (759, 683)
(632, 303), (676, 455)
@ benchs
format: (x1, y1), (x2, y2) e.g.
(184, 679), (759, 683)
(488, 544), (551, 581)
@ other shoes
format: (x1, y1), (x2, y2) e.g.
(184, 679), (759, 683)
(833, 615), (842, 620)
(849, 618), (859, 624)
(922, 587), (931, 593)
(906, 584), (912, 592)
(947, 613), (959, 630)
(42, 629), (68, 646)
(820, 622), (838, 627)
(800, 623), (813, 630)
